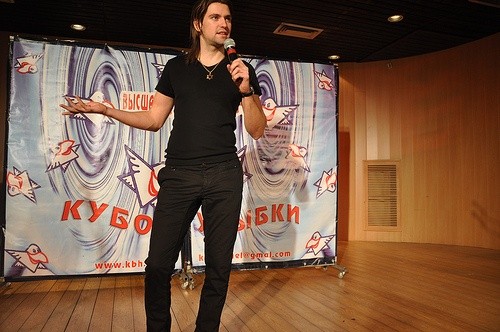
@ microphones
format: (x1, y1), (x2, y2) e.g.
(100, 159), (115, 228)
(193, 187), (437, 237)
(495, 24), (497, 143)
(223, 38), (242, 80)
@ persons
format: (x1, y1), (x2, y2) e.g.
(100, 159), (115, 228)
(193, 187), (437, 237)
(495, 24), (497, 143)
(59, 0), (266, 332)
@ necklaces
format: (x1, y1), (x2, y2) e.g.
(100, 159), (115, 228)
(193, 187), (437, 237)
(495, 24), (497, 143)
(197, 56), (225, 80)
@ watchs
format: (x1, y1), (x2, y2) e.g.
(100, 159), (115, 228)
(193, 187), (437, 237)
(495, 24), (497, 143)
(241, 86), (255, 97)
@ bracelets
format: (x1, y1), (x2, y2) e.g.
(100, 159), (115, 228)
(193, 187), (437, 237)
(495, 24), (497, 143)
(103, 105), (108, 115)
